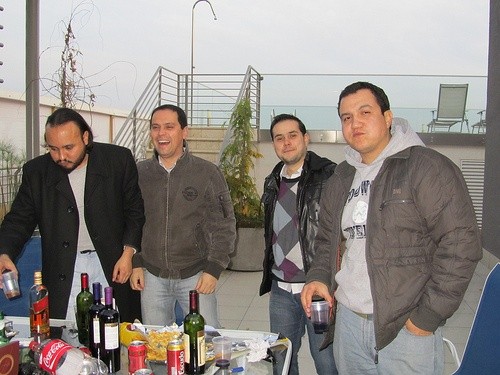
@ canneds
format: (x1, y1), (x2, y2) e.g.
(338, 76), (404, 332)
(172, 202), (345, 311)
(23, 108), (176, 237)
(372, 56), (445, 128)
(166, 340), (185, 375)
(127, 341), (154, 375)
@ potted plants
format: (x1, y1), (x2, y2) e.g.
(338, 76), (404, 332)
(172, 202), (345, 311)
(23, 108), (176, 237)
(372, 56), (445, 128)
(217, 98), (265, 273)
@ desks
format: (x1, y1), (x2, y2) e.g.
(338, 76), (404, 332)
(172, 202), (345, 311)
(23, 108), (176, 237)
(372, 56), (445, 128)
(2, 315), (293, 375)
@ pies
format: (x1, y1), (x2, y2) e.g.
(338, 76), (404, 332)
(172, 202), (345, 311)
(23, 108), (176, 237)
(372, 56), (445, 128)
(144, 331), (186, 360)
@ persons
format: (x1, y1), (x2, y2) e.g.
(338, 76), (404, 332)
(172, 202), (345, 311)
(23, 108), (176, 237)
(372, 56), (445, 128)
(299, 82), (482, 375)
(0, 109), (145, 325)
(259, 114), (338, 375)
(129, 105), (236, 329)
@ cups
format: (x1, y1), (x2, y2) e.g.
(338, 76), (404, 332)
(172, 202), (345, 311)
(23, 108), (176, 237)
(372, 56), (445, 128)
(309, 301), (331, 334)
(1, 271), (20, 300)
(212, 336), (232, 368)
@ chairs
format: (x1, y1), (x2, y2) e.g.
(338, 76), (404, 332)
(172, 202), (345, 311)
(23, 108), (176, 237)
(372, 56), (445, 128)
(0, 236), (43, 318)
(442, 263), (500, 375)
(427, 84), (469, 133)
(471, 110), (486, 134)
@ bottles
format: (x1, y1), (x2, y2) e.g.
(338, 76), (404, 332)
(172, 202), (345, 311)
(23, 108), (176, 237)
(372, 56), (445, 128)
(29, 339), (109, 375)
(0, 311), (9, 347)
(99, 287), (121, 373)
(76, 273), (93, 345)
(88, 282), (105, 362)
(184, 289), (206, 375)
(29, 271), (49, 341)
